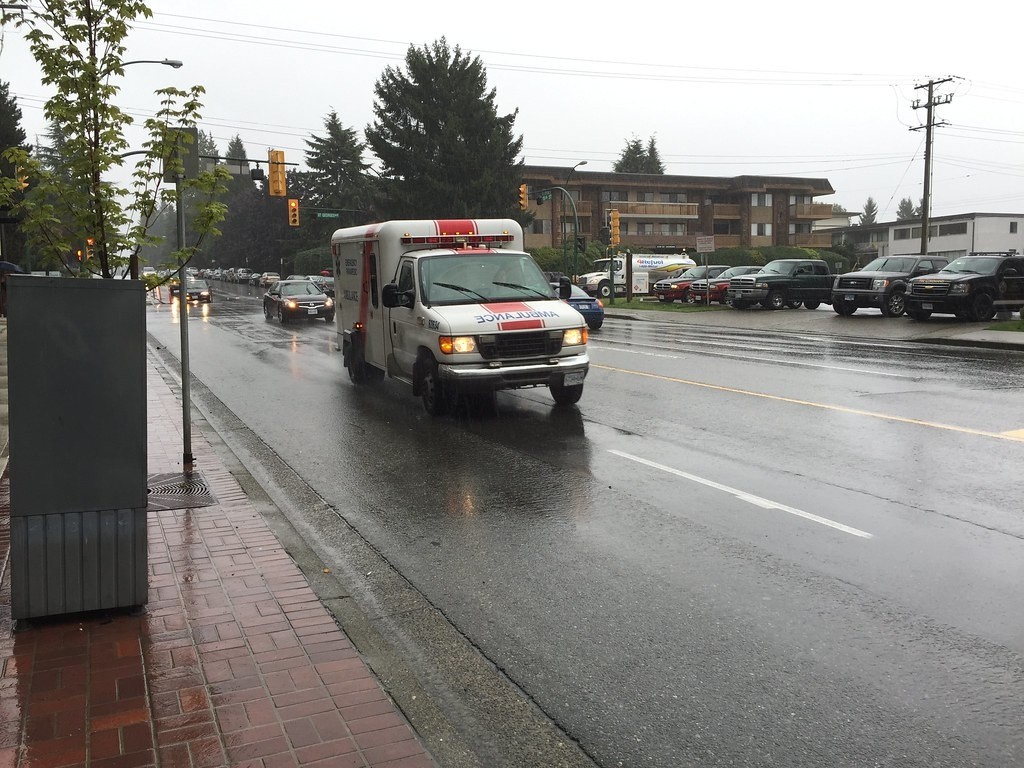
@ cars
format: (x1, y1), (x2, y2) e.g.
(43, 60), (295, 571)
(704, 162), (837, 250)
(168, 271), (213, 303)
(188, 267), (280, 288)
(549, 282), (605, 329)
(141, 266), (158, 277)
(263, 274), (335, 328)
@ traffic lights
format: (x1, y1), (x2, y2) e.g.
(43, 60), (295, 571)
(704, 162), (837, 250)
(288, 198), (299, 226)
(519, 184), (527, 210)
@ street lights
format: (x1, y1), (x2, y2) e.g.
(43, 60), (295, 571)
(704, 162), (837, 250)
(80, 59), (183, 277)
(563, 160), (588, 274)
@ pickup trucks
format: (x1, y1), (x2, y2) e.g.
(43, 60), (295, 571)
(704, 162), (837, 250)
(727, 258), (843, 310)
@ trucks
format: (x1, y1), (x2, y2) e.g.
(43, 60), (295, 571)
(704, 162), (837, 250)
(577, 252), (696, 299)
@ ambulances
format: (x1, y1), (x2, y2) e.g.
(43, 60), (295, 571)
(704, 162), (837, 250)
(331, 218), (590, 417)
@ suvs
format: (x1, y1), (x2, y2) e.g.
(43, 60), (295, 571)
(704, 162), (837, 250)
(690, 265), (765, 305)
(903, 248), (1024, 322)
(653, 265), (731, 304)
(831, 252), (950, 317)
